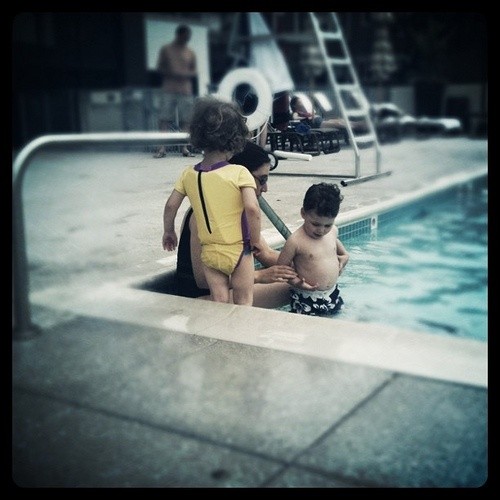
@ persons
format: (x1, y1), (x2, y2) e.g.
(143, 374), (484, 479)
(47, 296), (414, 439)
(155, 24), (201, 157)
(174, 142), (294, 309)
(160, 95), (263, 305)
(276, 181), (349, 317)
(290, 96), (367, 131)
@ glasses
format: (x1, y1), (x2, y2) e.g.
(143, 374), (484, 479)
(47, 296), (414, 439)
(253, 173), (268, 185)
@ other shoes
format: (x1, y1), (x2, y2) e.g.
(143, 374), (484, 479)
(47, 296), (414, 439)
(152, 150), (165, 158)
(182, 150), (195, 157)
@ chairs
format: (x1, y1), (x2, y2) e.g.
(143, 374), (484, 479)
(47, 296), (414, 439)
(265, 101), (462, 157)
